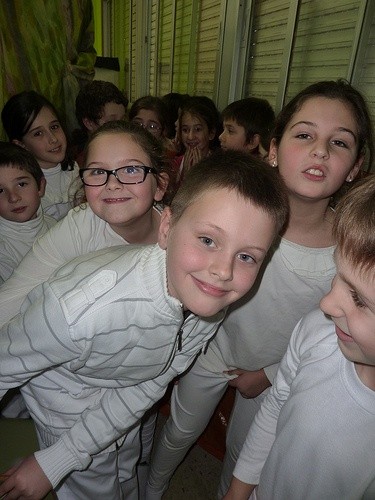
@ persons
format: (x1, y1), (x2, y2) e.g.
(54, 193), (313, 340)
(0, 76), (375, 500)
(0, 152), (287, 500)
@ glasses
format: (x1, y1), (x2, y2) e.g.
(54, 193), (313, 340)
(136, 121), (161, 132)
(79, 165), (159, 187)
